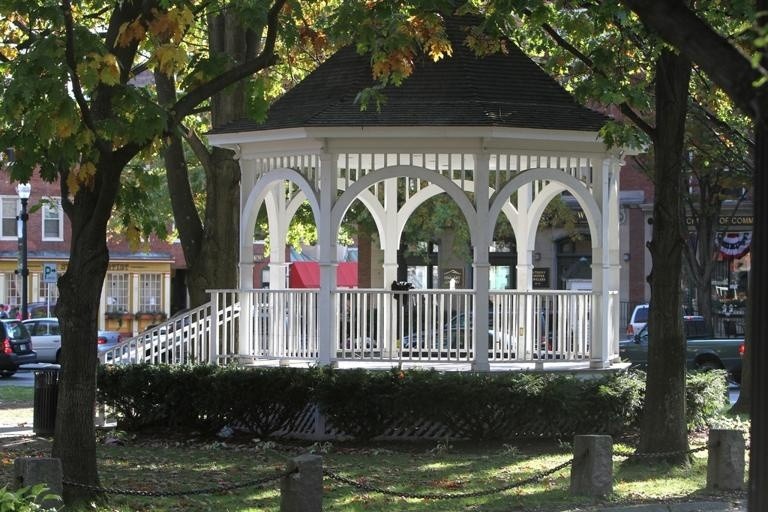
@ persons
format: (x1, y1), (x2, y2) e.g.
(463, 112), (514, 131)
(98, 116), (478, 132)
(0, 304), (30, 320)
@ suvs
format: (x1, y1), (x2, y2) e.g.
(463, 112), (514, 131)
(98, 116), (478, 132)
(0, 318), (37, 378)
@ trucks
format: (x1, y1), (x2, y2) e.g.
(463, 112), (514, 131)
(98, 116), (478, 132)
(619, 316), (745, 373)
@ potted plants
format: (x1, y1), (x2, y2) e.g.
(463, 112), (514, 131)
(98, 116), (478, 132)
(105, 311), (134, 327)
(136, 310), (167, 324)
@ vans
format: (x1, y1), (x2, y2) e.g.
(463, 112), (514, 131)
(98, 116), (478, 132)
(626, 304), (649, 337)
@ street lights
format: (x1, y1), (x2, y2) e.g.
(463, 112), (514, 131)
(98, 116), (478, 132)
(18, 182), (31, 320)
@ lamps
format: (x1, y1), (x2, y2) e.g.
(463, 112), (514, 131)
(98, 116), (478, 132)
(534, 253), (541, 261)
(623, 253), (631, 262)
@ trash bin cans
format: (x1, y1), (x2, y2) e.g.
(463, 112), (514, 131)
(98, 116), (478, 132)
(32, 367), (62, 436)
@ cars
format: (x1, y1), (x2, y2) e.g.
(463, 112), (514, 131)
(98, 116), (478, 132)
(21, 317), (125, 365)
